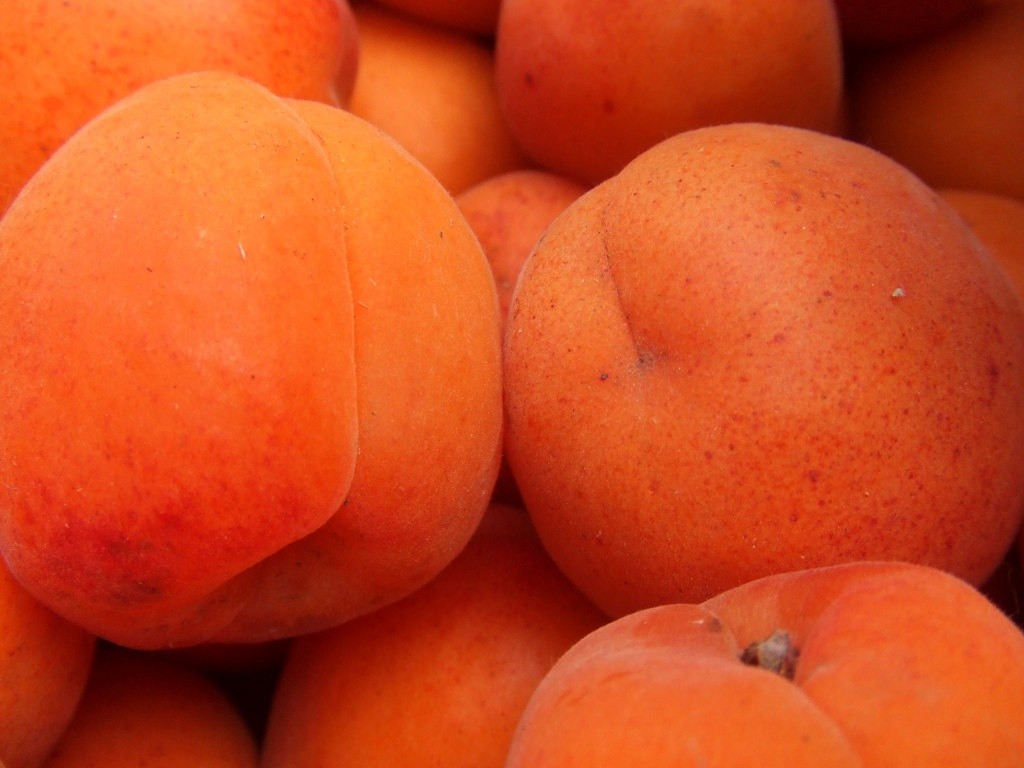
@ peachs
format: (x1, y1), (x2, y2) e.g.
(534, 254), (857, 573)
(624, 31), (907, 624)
(0, 0), (1024, 768)
